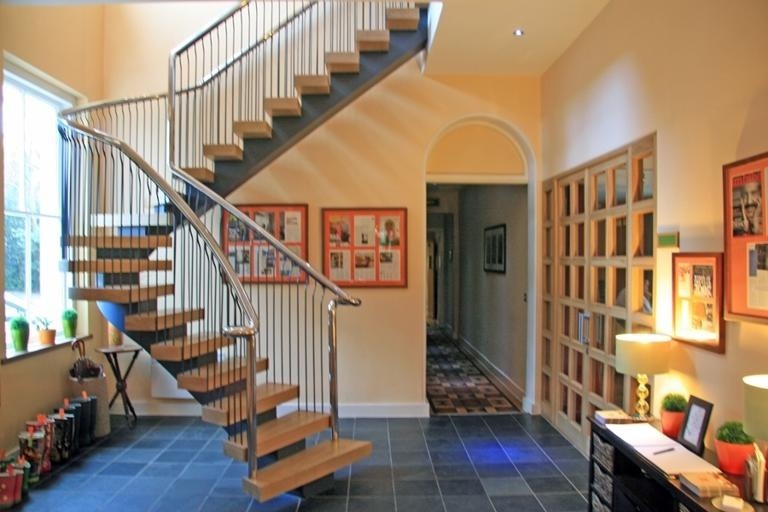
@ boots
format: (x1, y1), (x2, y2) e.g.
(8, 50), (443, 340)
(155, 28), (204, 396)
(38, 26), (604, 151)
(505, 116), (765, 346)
(1, 473), (16, 510)
(5, 468), (23, 504)
(70, 399), (93, 446)
(48, 415), (73, 460)
(26, 421), (52, 472)
(7, 463), (31, 495)
(19, 435), (45, 484)
(54, 408), (81, 456)
(92, 397), (98, 442)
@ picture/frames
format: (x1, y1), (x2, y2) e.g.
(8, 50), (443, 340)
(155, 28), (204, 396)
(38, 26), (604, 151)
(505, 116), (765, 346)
(671, 252), (725, 355)
(722, 151), (768, 327)
(484, 223), (506, 275)
(678, 394), (714, 457)
(220, 203), (309, 285)
(321, 207), (408, 288)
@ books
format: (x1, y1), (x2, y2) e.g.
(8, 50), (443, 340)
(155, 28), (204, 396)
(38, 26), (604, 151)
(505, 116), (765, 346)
(594, 409), (633, 426)
(604, 422), (723, 475)
(679, 471), (740, 497)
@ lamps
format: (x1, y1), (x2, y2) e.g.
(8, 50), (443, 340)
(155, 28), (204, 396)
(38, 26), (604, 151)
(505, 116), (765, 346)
(615, 333), (672, 423)
(742, 374), (768, 442)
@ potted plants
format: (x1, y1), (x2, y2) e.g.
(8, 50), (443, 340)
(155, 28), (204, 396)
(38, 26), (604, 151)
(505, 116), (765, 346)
(714, 420), (757, 475)
(32, 317), (56, 345)
(660, 393), (688, 438)
(61, 309), (78, 338)
(10, 317), (29, 352)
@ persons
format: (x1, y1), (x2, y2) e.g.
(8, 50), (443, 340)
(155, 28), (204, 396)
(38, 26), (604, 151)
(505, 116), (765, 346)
(615, 276), (652, 331)
(739, 181), (762, 234)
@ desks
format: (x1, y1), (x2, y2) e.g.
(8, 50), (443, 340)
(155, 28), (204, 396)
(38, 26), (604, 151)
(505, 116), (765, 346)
(94, 345), (143, 431)
(585, 415), (768, 512)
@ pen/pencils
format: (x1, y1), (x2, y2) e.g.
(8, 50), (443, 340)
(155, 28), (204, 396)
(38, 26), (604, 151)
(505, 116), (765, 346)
(654, 447), (674, 454)
